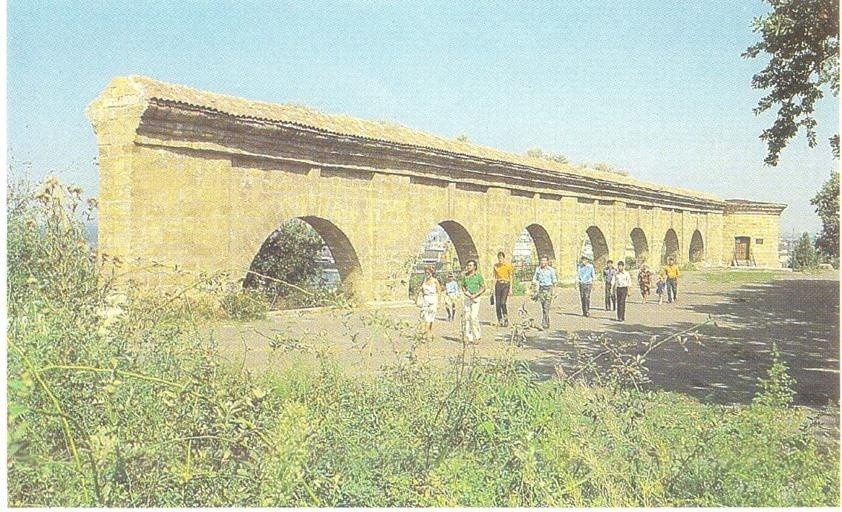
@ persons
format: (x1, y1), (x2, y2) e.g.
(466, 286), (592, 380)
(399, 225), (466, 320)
(445, 274), (459, 321)
(491, 253), (513, 327)
(461, 260), (487, 343)
(531, 256), (557, 328)
(602, 260), (617, 310)
(575, 256), (596, 317)
(417, 267), (441, 334)
(638, 256), (680, 306)
(609, 261), (631, 322)
